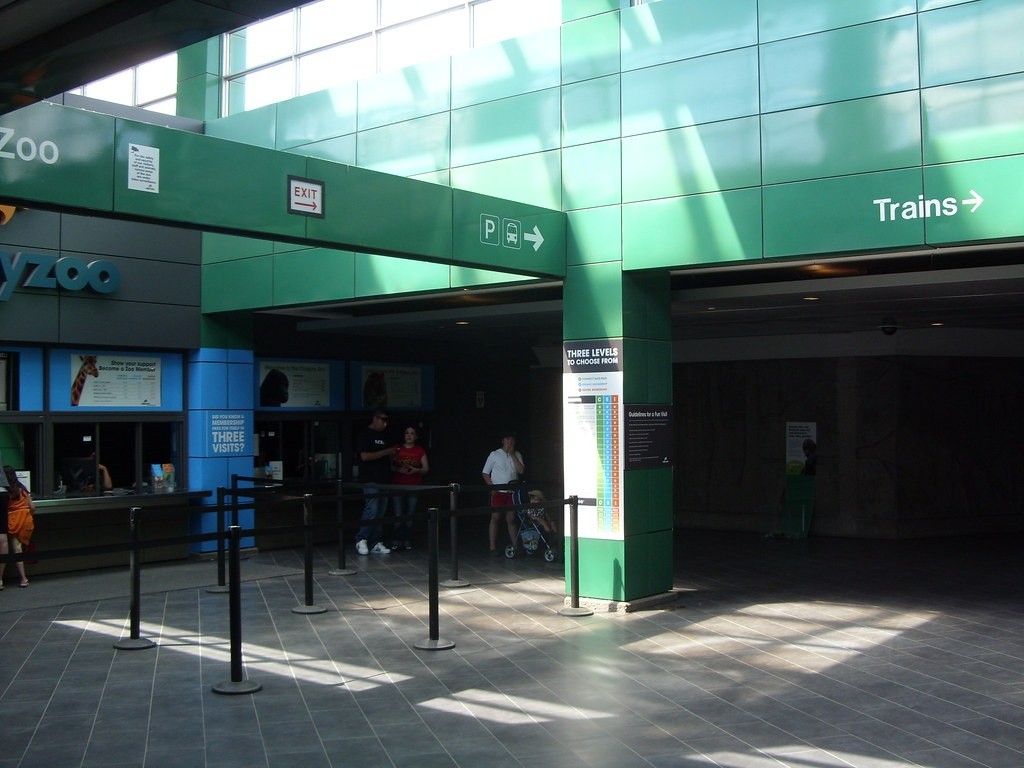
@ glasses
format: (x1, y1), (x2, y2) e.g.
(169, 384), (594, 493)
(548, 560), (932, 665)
(377, 416), (388, 421)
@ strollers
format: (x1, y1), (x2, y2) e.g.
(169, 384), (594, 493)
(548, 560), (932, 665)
(503, 480), (561, 562)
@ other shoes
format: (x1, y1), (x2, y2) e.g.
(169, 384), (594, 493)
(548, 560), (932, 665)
(0, 580), (5, 590)
(488, 549), (500, 556)
(391, 545), (399, 550)
(20, 582), (29, 587)
(404, 544), (412, 550)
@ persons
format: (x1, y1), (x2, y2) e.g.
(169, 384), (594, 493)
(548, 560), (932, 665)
(3, 465), (36, 587)
(481, 430), (526, 558)
(81, 447), (113, 491)
(524, 489), (558, 535)
(0, 451), (10, 590)
(799, 438), (817, 476)
(387, 426), (429, 551)
(354, 409), (402, 554)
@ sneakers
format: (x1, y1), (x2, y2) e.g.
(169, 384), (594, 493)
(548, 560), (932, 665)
(369, 542), (391, 554)
(356, 539), (369, 555)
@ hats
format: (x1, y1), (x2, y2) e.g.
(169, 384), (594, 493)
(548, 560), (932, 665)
(528, 489), (544, 502)
